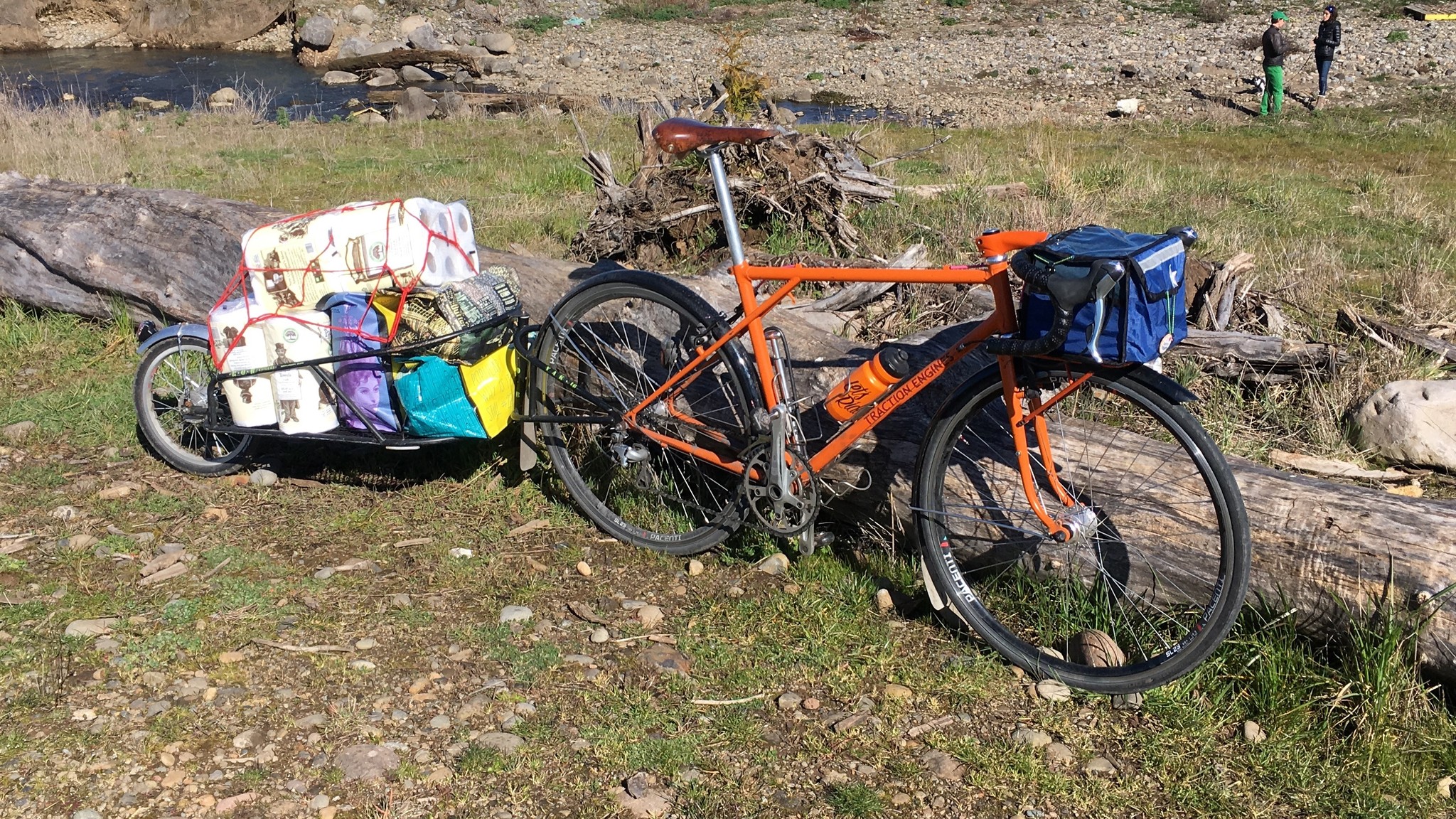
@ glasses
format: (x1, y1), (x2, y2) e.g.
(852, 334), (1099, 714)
(1323, 12), (1329, 15)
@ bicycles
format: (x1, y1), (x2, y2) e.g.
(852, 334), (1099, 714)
(510, 118), (1249, 695)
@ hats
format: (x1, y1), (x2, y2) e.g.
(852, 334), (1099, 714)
(1272, 11), (1289, 21)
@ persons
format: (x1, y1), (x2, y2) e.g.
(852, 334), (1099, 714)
(1260, 11), (1291, 115)
(1313, 6), (1341, 110)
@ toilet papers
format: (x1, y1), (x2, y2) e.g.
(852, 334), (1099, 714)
(262, 310), (342, 437)
(212, 295), (276, 429)
(238, 196), (480, 291)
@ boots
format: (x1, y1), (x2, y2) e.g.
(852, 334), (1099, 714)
(1311, 95), (1326, 110)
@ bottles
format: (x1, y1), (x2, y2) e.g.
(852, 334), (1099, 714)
(825, 347), (909, 423)
